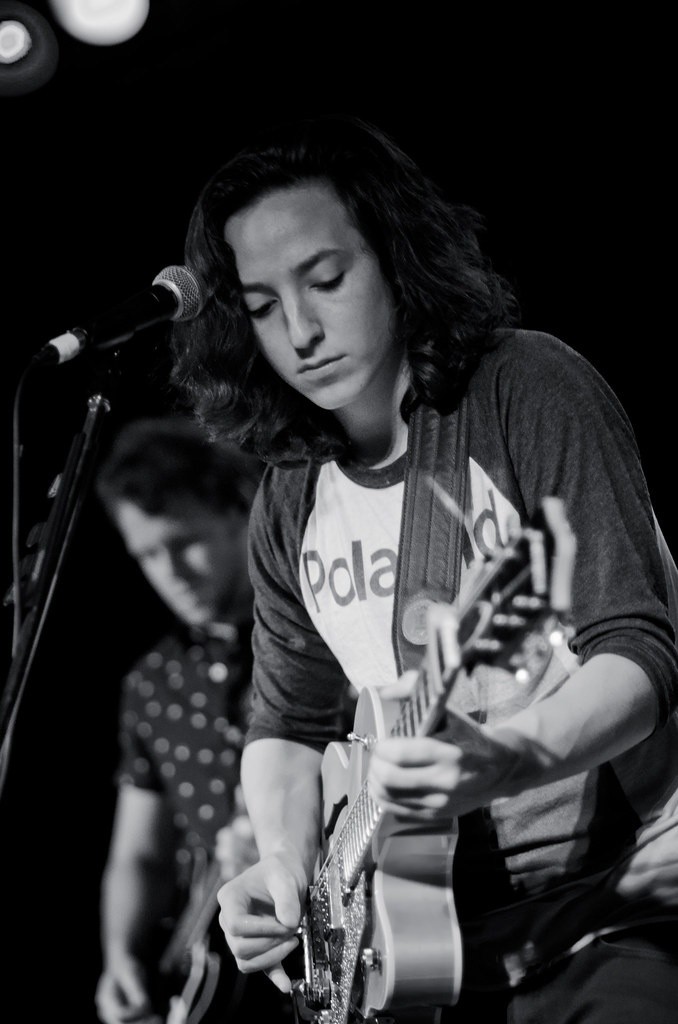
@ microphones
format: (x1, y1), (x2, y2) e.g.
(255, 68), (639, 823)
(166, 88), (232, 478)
(32, 265), (210, 365)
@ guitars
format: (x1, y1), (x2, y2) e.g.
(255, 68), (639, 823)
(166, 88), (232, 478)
(282, 496), (578, 1024)
(148, 785), (226, 1024)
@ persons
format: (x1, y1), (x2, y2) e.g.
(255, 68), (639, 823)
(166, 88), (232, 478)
(95, 417), (261, 1024)
(171, 129), (678, 1024)
